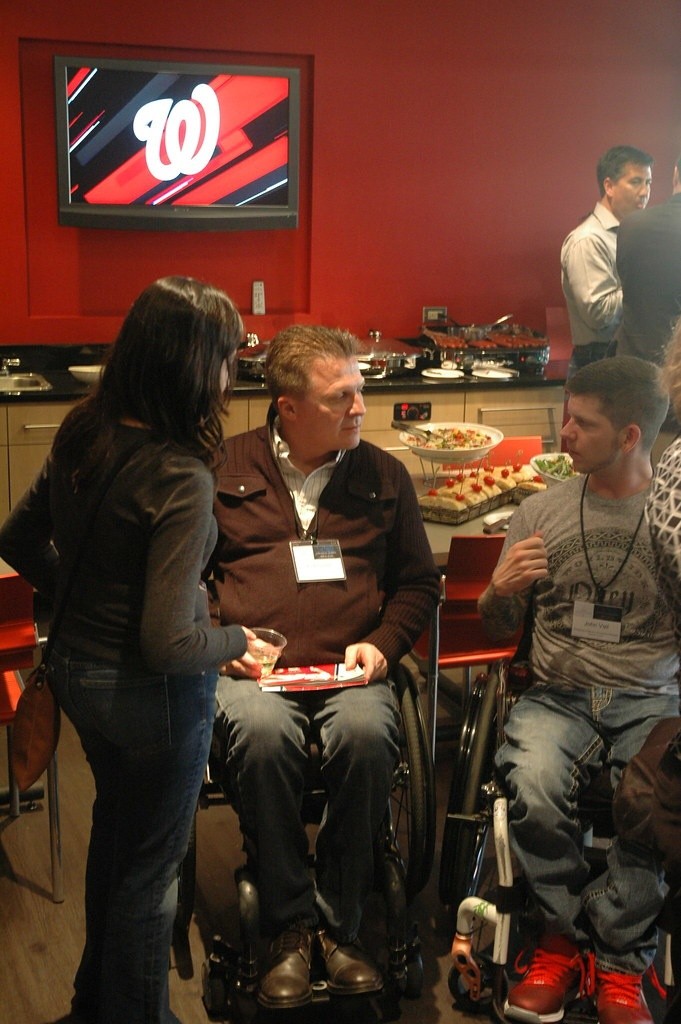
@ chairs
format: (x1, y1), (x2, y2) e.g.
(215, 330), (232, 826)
(446, 436), (545, 468)
(0, 574), (68, 904)
(410, 535), (520, 764)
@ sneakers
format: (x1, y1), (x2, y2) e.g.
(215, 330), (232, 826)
(503, 931), (586, 1023)
(586, 953), (668, 1024)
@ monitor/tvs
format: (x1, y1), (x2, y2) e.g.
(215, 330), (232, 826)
(52, 54), (299, 231)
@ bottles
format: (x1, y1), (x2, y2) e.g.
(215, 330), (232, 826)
(366, 329), (386, 380)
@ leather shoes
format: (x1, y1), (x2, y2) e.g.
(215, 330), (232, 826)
(261, 929), (317, 1008)
(317, 929), (385, 994)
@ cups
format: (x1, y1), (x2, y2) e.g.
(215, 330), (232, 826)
(246, 628), (287, 678)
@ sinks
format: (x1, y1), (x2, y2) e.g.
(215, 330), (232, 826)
(0, 375), (42, 389)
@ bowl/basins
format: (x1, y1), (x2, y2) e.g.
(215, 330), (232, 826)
(356, 362), (370, 374)
(529, 453), (581, 487)
(68, 365), (105, 383)
(237, 349), (266, 380)
(399, 423), (504, 464)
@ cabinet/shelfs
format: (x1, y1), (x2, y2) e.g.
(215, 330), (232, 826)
(352, 392), (463, 474)
(7, 404), (84, 510)
(464, 388), (564, 460)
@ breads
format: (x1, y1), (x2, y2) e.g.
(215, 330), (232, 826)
(416, 464), (546, 510)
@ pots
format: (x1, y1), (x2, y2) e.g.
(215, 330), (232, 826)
(383, 353), (420, 376)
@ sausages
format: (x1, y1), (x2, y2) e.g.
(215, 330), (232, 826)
(433, 333), (549, 348)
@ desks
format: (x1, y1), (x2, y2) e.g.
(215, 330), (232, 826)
(412, 473), (553, 567)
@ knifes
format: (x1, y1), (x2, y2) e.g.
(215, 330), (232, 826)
(483, 514), (514, 534)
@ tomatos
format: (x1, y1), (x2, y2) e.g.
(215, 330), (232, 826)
(483, 476), (494, 485)
(445, 477), (454, 488)
(513, 463), (521, 472)
(533, 476), (543, 482)
(471, 484), (482, 491)
(428, 488), (438, 496)
(470, 472), (476, 477)
(455, 495), (464, 500)
(484, 465), (494, 471)
(501, 468), (509, 477)
(457, 474), (466, 481)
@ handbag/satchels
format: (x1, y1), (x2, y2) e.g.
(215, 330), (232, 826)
(11, 666), (58, 792)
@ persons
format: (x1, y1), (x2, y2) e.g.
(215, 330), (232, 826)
(646, 322), (681, 703)
(0, 275), (263, 1024)
(210, 324), (443, 1009)
(560, 146), (654, 397)
(476, 355), (681, 1024)
(615, 154), (681, 471)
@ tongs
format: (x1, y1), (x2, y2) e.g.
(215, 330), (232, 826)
(391, 421), (446, 446)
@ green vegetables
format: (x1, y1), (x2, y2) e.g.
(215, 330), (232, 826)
(534, 454), (576, 481)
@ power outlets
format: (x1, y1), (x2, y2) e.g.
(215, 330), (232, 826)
(423, 307), (447, 323)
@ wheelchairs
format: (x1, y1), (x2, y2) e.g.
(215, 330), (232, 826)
(173, 648), (440, 1024)
(434, 659), (681, 1024)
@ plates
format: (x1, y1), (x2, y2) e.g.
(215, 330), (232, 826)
(483, 512), (514, 530)
(471, 370), (513, 379)
(421, 368), (465, 379)
